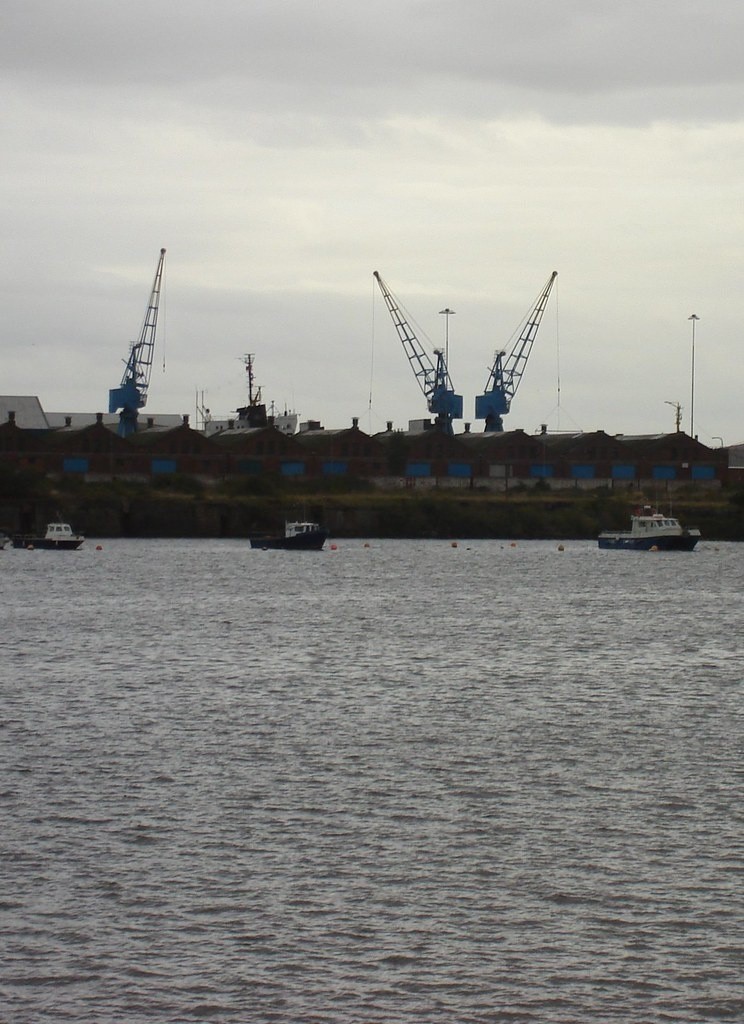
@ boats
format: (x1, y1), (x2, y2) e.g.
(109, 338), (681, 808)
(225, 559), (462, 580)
(10, 522), (87, 551)
(597, 504), (702, 551)
(250, 519), (331, 553)
(0, 533), (10, 549)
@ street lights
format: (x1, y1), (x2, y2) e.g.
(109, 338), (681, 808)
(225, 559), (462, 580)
(687, 314), (701, 441)
(438, 306), (457, 388)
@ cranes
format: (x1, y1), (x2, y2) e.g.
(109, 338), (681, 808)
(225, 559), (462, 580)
(103, 248), (167, 439)
(370, 269), (560, 434)
